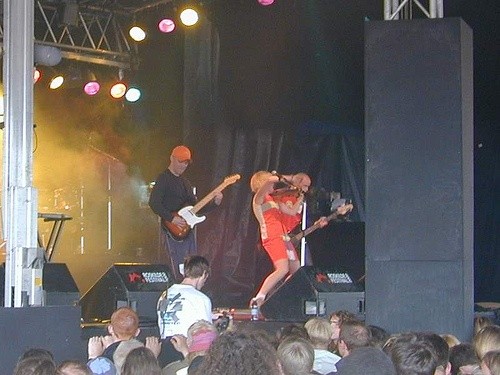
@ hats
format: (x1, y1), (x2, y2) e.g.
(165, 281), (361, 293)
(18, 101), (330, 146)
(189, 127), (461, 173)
(171, 145), (192, 161)
(186, 320), (218, 354)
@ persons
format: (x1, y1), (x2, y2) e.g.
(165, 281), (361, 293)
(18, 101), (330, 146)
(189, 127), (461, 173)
(288, 173), (328, 267)
(250, 171), (303, 321)
(14, 254), (500, 375)
(148, 145), (222, 284)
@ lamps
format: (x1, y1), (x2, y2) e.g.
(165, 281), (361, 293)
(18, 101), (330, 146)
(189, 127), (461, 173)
(80, 67), (100, 97)
(156, 4), (177, 34)
(38, 65), (65, 90)
(105, 72), (127, 99)
(127, 15), (150, 42)
(125, 78), (143, 104)
(34, 66), (42, 85)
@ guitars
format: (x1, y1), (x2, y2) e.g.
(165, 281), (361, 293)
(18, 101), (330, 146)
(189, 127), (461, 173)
(160, 173), (241, 243)
(258, 204), (355, 262)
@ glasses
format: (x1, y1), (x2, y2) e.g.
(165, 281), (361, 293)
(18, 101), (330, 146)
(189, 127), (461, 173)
(329, 320), (338, 325)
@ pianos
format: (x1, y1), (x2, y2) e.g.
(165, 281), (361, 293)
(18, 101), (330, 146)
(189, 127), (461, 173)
(34, 213), (73, 264)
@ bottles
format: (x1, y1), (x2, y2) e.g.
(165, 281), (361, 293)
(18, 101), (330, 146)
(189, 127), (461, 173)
(250, 298), (258, 321)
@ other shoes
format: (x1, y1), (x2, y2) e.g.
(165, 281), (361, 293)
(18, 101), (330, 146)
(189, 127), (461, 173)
(248, 297), (266, 321)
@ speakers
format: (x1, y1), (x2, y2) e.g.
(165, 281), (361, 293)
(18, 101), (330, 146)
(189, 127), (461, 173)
(1, 262), (177, 322)
(258, 263), (365, 321)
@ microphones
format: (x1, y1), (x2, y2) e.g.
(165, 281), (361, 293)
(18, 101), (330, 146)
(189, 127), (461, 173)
(271, 169), (287, 182)
